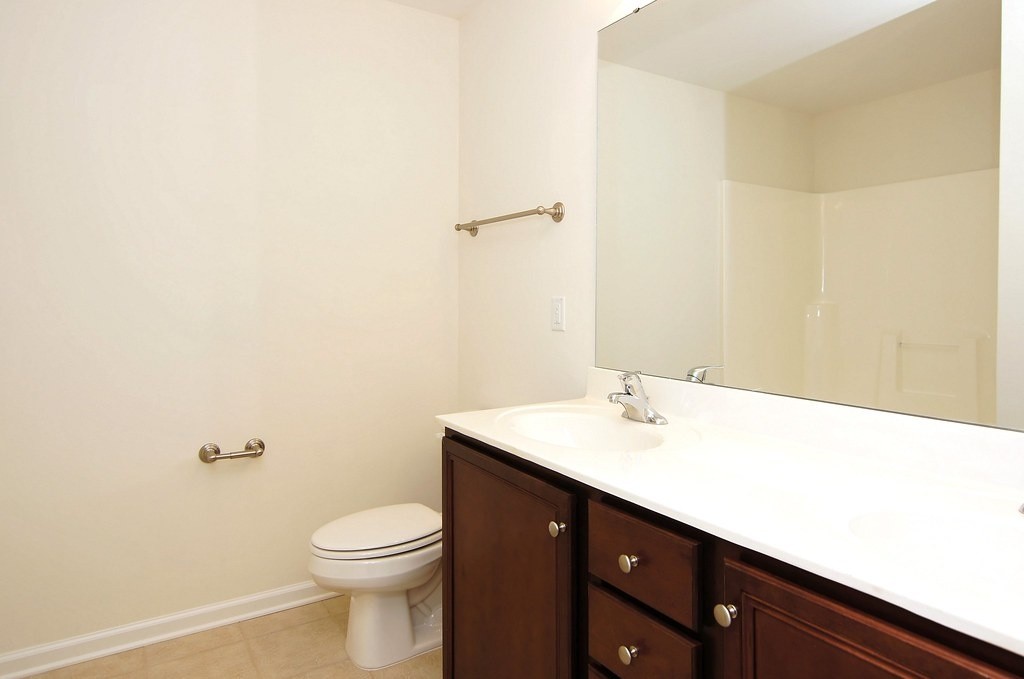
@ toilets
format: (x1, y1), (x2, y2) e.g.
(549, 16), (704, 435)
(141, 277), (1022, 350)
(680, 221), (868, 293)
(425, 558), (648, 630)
(308, 502), (443, 671)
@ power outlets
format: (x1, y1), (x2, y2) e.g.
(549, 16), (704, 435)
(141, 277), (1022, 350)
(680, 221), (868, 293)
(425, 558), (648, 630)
(551, 297), (565, 331)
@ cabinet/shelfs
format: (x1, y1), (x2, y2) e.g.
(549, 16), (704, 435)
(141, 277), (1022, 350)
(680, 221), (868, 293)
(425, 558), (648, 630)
(716, 537), (1024, 679)
(584, 485), (716, 678)
(441, 427), (584, 679)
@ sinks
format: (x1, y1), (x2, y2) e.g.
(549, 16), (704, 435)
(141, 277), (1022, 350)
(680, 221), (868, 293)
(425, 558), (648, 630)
(497, 406), (666, 450)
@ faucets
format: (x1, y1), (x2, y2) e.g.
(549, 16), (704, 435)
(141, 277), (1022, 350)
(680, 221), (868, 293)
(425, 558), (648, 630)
(607, 371), (667, 426)
(685, 366), (726, 385)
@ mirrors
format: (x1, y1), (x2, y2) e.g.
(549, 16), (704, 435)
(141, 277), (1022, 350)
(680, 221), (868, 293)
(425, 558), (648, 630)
(595, 1), (1024, 434)
